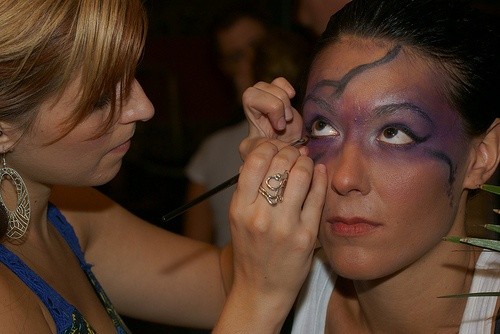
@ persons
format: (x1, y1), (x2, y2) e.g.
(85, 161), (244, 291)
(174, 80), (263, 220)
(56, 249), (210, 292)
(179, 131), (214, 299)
(0, 0), (328, 334)
(280, 0), (500, 334)
(185, 22), (319, 250)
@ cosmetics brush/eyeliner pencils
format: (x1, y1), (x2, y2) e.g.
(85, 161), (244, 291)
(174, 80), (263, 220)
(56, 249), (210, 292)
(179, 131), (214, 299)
(162, 135), (310, 222)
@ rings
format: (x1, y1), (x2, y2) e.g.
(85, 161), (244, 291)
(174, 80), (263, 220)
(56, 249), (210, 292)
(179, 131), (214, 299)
(258, 170), (289, 205)
